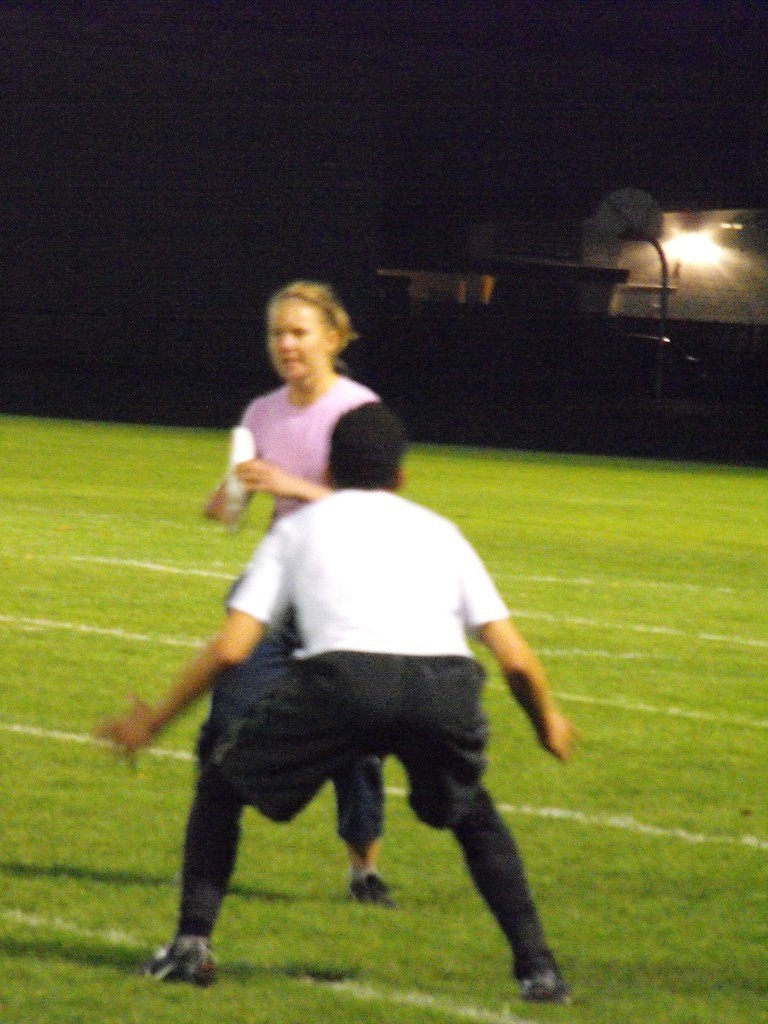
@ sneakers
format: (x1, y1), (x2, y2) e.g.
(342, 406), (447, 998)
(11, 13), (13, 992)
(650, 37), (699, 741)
(514, 946), (574, 1005)
(349, 873), (395, 910)
(141, 941), (220, 993)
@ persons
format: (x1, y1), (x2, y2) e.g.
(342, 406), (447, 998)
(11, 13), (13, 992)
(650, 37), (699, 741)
(101, 403), (575, 1003)
(202, 280), (396, 905)
